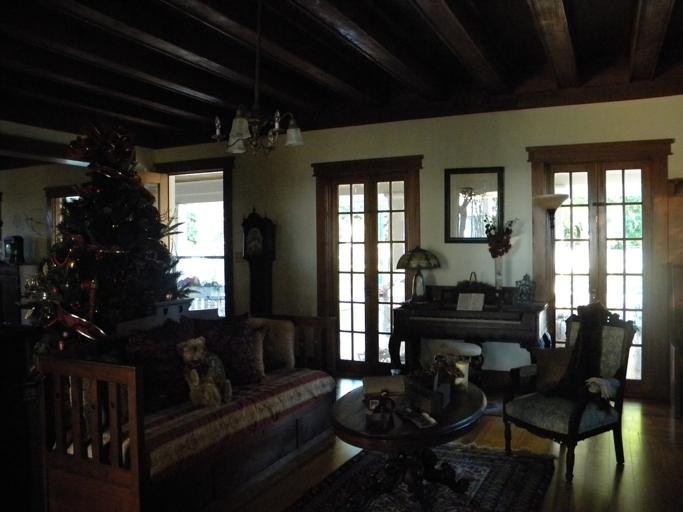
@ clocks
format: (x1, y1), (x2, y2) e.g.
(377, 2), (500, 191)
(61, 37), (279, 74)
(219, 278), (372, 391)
(240, 212), (273, 260)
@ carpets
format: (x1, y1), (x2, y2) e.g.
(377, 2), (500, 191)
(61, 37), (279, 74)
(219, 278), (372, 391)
(282, 440), (554, 511)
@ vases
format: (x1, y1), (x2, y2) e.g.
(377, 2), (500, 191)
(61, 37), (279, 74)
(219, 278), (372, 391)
(494, 256), (506, 291)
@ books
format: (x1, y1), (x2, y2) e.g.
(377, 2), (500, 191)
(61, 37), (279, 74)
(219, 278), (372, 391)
(456, 293), (486, 312)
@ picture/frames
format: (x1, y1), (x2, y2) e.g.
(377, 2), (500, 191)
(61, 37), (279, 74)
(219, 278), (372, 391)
(444, 166), (504, 244)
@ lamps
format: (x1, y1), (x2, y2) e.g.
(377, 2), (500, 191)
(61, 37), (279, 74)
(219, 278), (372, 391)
(533, 193), (567, 349)
(396, 245), (441, 309)
(210, 3), (304, 156)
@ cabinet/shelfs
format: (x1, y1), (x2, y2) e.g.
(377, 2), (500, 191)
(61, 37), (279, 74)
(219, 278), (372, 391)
(0, 265), (38, 328)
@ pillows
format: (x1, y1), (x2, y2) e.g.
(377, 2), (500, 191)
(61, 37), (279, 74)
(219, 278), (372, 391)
(44, 311), (297, 424)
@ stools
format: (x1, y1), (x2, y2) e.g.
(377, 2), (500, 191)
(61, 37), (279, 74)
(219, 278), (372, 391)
(441, 342), (482, 363)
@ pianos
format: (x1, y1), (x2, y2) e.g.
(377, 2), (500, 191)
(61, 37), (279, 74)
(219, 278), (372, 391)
(389, 286), (544, 377)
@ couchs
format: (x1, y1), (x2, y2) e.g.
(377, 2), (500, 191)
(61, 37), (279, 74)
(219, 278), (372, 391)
(32, 317), (336, 511)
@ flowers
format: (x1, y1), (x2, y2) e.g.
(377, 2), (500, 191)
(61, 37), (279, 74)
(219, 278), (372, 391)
(482, 218), (518, 259)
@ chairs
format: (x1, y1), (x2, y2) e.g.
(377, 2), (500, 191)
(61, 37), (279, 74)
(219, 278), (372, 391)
(502, 301), (636, 484)
